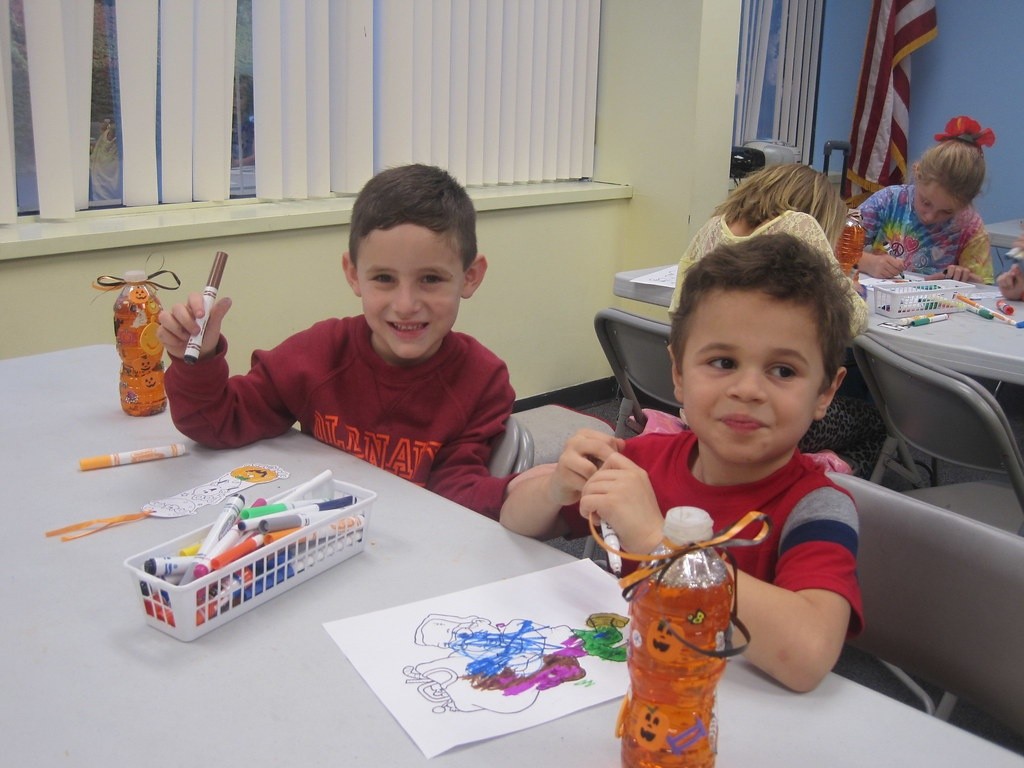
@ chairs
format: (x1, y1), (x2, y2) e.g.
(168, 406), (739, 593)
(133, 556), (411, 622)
(852, 331), (1024, 537)
(825, 470), (1024, 757)
(593, 307), (683, 438)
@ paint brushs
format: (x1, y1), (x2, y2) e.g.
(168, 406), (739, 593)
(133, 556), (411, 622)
(880, 292), (1024, 328)
(881, 240), (905, 280)
(183, 250), (227, 366)
(79, 443), (187, 471)
(996, 299), (1015, 315)
(138, 467), (367, 629)
(595, 458), (622, 580)
(896, 312), (949, 326)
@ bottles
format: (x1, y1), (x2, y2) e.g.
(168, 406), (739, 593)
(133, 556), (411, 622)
(834, 208), (866, 277)
(112, 270), (168, 417)
(621, 507), (737, 768)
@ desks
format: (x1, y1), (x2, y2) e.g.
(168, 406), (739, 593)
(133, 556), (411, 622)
(0, 345), (1024, 768)
(612, 264), (1024, 487)
(983, 218), (1024, 249)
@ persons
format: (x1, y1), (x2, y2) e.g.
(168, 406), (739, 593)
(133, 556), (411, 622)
(499, 233), (865, 691)
(157, 164), (558, 520)
(669, 165), (890, 478)
(229, 73), (254, 167)
(996, 264), (1023, 471)
(88, 122), (120, 198)
(853, 116), (997, 286)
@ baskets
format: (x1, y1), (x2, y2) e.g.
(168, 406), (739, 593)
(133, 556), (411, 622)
(873, 278), (975, 319)
(122, 469), (377, 643)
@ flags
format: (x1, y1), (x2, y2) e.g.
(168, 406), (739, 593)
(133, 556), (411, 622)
(844, 0), (938, 210)
